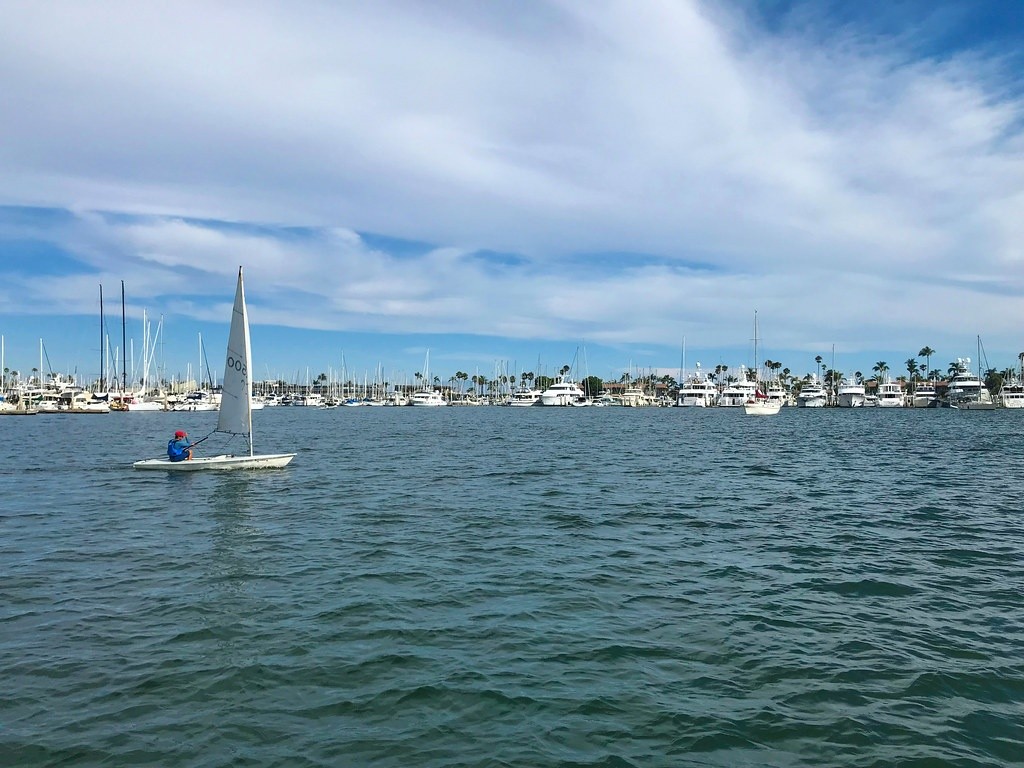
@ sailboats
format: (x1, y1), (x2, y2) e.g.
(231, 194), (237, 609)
(128, 263), (298, 473)
(0, 277), (1024, 417)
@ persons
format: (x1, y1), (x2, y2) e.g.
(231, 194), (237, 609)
(168, 430), (192, 462)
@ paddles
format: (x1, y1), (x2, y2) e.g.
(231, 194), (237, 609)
(183, 437), (209, 450)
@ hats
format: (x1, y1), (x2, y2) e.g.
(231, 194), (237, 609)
(175, 431), (187, 436)
